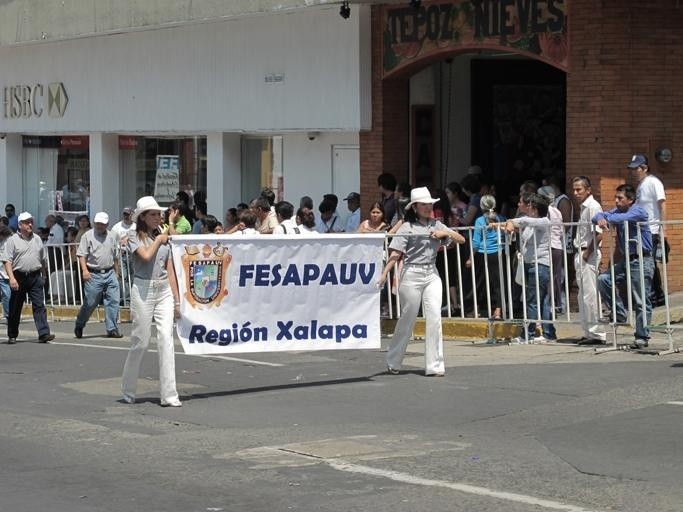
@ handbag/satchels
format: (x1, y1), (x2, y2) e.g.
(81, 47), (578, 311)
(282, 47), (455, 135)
(513, 252), (528, 289)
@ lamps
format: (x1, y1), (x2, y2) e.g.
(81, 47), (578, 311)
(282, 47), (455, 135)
(338, 1), (351, 20)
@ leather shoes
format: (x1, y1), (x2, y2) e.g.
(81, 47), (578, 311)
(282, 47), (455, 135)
(73, 326), (84, 338)
(572, 336), (607, 346)
(38, 334), (55, 343)
(107, 329), (123, 338)
(7, 336), (16, 344)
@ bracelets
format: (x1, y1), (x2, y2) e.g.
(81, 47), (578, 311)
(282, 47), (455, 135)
(176, 303), (180, 305)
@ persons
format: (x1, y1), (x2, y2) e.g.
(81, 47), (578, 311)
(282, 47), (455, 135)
(1, 204), (91, 344)
(376, 187), (465, 376)
(164, 188), (360, 234)
(359, 202), (388, 232)
(592, 185), (654, 348)
(121, 196), (182, 406)
(73, 212), (123, 338)
(110, 207), (136, 306)
(378, 173), (412, 234)
(572, 176), (607, 344)
(628, 154), (664, 307)
(430, 166), (573, 344)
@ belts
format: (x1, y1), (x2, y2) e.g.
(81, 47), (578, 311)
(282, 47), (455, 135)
(618, 252), (653, 262)
(87, 264), (115, 275)
(573, 247), (588, 254)
(132, 278), (171, 288)
(405, 263), (437, 269)
(14, 269), (41, 277)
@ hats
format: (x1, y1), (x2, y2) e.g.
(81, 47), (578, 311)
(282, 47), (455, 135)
(403, 186), (441, 210)
(17, 211), (35, 223)
(120, 206), (135, 215)
(94, 211), (109, 225)
(131, 195), (170, 223)
(342, 191), (360, 201)
(626, 153), (648, 170)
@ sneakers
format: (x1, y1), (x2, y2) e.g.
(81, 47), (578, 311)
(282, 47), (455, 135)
(634, 337), (648, 350)
(165, 400), (183, 407)
(120, 390), (136, 405)
(386, 363), (399, 376)
(430, 371), (445, 377)
(533, 335), (557, 345)
(450, 305), (462, 316)
(597, 314), (627, 324)
(510, 336), (533, 344)
